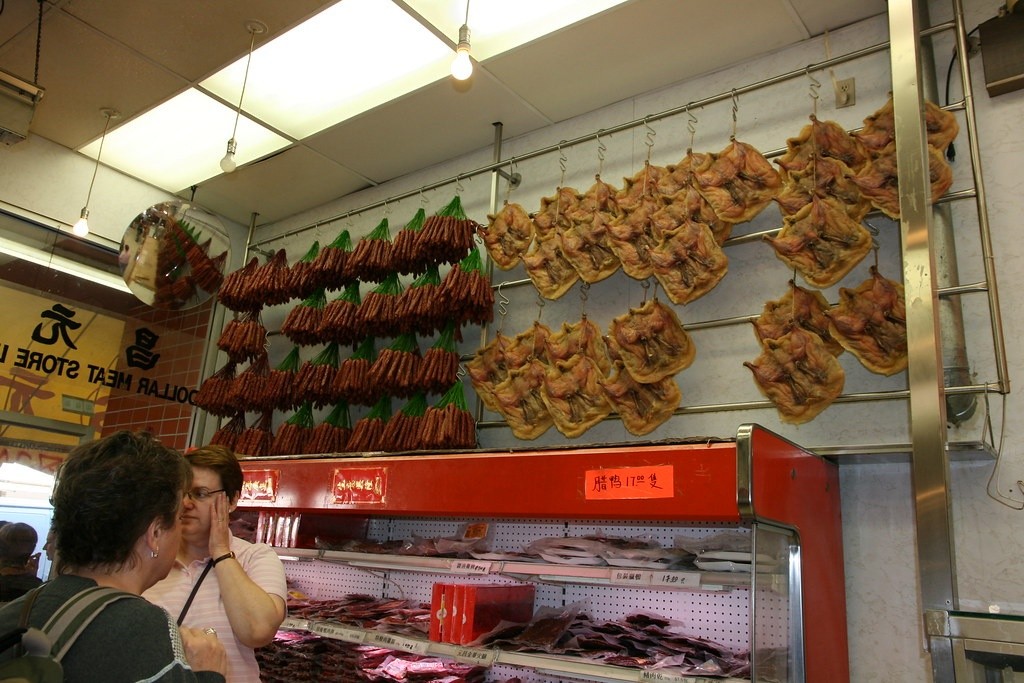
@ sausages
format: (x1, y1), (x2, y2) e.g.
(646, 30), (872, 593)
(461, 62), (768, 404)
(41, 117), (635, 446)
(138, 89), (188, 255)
(194, 216), (496, 453)
(152, 223), (223, 310)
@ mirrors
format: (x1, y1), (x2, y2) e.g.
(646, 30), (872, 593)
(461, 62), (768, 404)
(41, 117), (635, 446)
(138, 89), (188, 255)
(116, 199), (233, 314)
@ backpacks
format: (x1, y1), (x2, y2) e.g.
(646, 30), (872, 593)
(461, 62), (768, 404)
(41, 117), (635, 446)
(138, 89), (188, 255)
(0, 580), (147, 683)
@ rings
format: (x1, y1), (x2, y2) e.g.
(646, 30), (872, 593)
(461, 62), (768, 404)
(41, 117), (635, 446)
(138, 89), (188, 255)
(218, 519), (224, 523)
(205, 627), (217, 635)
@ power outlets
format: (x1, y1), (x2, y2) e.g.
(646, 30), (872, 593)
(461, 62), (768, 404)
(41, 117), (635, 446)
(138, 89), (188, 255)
(835, 78), (856, 109)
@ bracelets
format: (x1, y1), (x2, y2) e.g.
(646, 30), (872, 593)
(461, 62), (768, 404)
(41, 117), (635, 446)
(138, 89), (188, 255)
(213, 551), (235, 568)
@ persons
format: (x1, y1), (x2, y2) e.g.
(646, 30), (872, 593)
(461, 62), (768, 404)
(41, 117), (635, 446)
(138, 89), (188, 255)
(0, 430), (288, 683)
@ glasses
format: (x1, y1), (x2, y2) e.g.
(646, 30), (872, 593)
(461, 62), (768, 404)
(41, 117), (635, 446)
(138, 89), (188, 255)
(181, 488), (225, 502)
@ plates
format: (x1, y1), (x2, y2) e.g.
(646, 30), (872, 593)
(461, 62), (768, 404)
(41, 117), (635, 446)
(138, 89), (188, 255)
(466, 547), (777, 573)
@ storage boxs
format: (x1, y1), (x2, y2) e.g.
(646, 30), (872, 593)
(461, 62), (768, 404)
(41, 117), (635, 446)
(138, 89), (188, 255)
(255, 511), (352, 548)
(428, 582), (535, 646)
